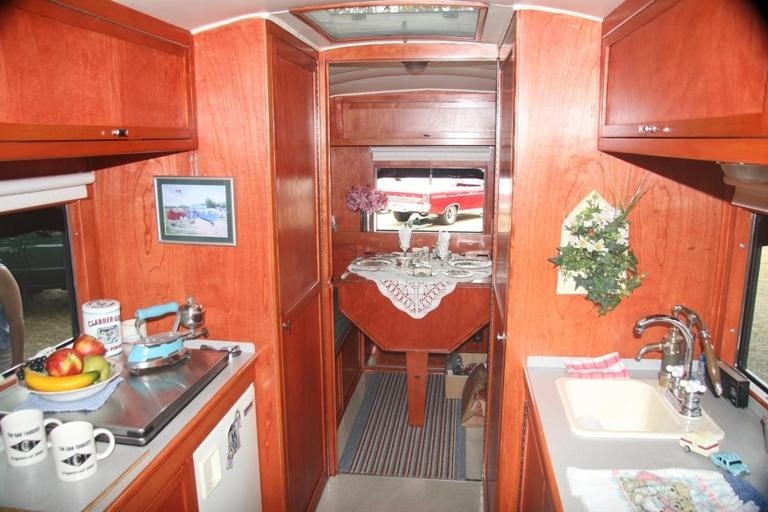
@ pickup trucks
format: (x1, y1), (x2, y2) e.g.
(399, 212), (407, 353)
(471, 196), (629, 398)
(379, 180), (484, 223)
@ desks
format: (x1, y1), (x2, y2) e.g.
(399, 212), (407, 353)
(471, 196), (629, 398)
(335, 254), (491, 427)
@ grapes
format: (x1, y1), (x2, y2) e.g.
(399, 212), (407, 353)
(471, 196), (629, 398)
(17, 356), (47, 380)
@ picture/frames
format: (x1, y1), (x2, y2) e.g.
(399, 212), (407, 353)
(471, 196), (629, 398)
(152, 176), (237, 246)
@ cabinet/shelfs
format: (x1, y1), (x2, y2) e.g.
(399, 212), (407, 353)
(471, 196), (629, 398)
(598, 1), (768, 164)
(511, 372), (561, 512)
(483, 11), (728, 512)
(0, 1), (199, 179)
(85, 19), (330, 512)
(101, 366), (266, 512)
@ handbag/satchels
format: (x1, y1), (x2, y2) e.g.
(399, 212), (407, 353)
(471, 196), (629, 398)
(460, 362), (487, 426)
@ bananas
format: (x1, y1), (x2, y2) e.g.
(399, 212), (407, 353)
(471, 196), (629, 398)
(23, 367), (101, 393)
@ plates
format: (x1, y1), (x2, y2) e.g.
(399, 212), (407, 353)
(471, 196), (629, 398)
(353, 252), (493, 279)
(19, 362), (122, 402)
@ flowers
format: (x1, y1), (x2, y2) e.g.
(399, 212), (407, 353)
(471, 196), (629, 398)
(548, 172), (648, 316)
(345, 185), (388, 214)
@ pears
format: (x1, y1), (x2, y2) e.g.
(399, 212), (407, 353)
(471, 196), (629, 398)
(82, 356), (111, 381)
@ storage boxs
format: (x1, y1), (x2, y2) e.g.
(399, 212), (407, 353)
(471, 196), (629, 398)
(445, 353), (487, 400)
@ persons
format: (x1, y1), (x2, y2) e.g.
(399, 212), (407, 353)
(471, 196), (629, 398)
(0, 262), (26, 372)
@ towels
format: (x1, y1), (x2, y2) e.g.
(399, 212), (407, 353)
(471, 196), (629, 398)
(565, 352), (630, 380)
(613, 470), (768, 512)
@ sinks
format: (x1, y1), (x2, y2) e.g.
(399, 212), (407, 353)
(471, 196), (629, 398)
(554, 376), (683, 439)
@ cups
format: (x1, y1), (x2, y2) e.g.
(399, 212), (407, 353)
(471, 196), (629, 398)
(48, 421), (117, 484)
(0, 407), (63, 468)
(119, 318), (147, 345)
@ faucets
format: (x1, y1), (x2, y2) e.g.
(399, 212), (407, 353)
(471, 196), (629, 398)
(635, 342), (667, 362)
(634, 314), (695, 401)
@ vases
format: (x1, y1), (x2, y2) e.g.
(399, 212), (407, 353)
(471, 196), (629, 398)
(360, 209), (374, 233)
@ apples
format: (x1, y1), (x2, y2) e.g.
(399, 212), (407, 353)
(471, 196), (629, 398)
(72, 335), (106, 360)
(46, 348), (84, 377)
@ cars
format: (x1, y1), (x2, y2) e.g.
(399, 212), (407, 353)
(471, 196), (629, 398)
(0, 228), (68, 297)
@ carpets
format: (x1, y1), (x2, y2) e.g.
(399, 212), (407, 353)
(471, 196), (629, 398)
(338, 371), (466, 480)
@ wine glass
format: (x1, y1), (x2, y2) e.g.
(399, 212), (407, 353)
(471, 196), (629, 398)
(400, 228), (411, 256)
(437, 240), (449, 262)
